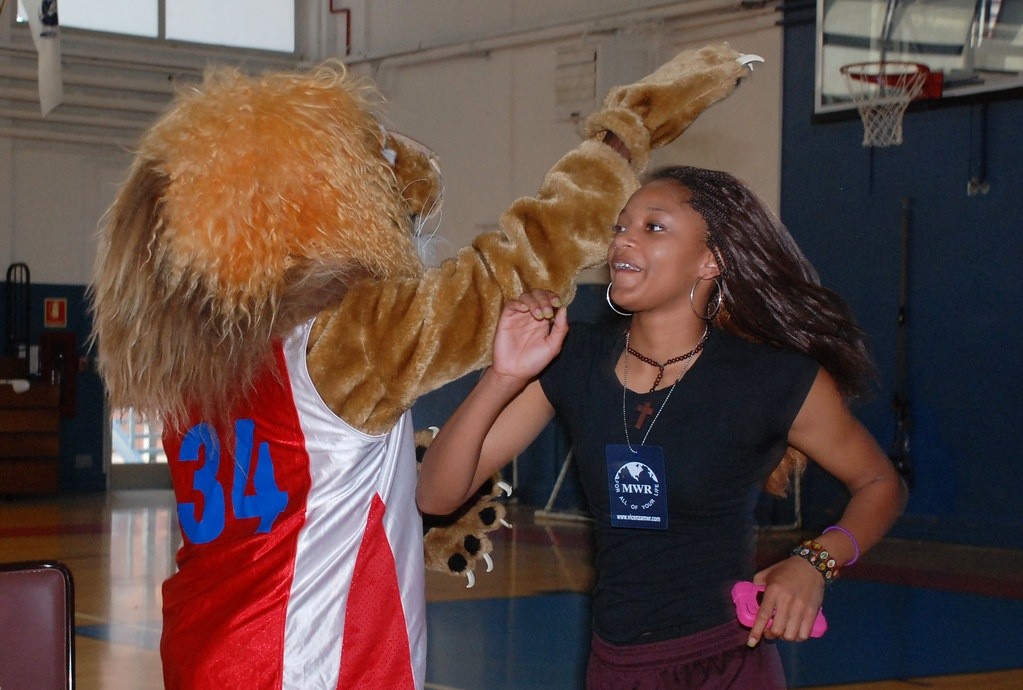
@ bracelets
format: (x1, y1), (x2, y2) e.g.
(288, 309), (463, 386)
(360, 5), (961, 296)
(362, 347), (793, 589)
(789, 525), (858, 582)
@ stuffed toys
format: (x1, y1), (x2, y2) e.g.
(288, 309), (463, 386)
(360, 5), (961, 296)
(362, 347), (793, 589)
(86, 38), (766, 690)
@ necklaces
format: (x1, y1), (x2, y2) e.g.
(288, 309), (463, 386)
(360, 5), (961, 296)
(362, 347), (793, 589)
(625, 325), (708, 390)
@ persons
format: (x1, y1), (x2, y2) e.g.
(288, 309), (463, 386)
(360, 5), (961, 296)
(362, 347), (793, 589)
(418, 166), (908, 690)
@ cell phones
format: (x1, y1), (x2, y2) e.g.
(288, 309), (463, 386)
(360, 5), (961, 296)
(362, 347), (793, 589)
(731, 581), (828, 638)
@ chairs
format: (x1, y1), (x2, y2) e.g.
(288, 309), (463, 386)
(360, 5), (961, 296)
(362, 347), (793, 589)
(0, 559), (77, 690)
